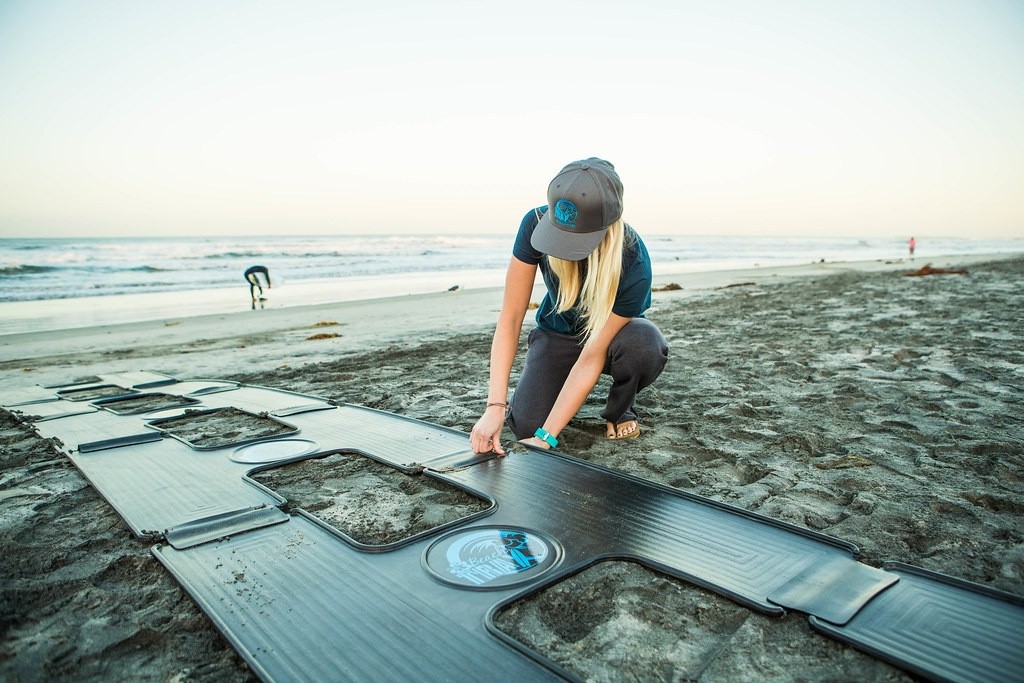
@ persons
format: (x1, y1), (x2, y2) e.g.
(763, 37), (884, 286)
(244, 265), (272, 301)
(909, 237), (915, 253)
(469, 157), (669, 454)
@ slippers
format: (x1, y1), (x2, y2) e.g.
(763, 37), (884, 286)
(605, 419), (640, 440)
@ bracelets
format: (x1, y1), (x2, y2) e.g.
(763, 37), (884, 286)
(535, 427), (558, 449)
(486, 402), (506, 408)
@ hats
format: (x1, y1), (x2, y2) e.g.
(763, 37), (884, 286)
(529, 157), (625, 261)
